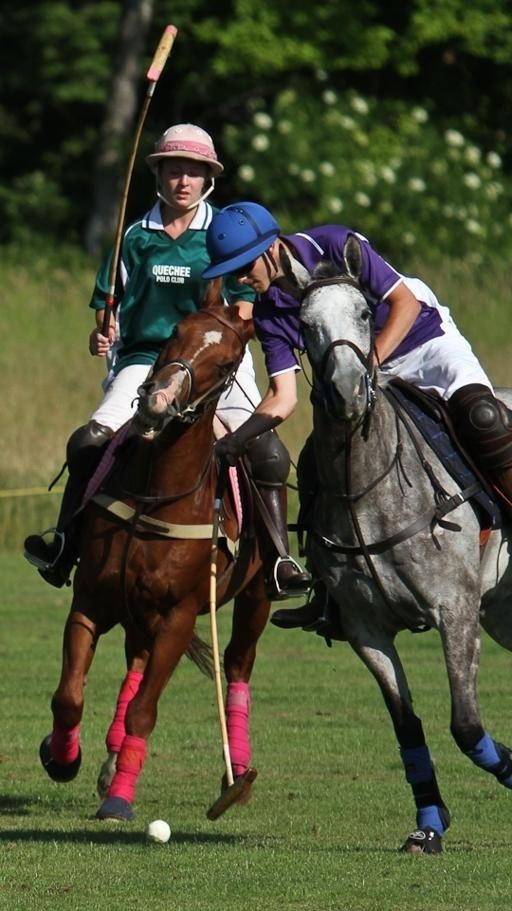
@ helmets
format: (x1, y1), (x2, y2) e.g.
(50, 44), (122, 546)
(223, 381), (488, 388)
(202, 201), (281, 280)
(146, 124), (224, 179)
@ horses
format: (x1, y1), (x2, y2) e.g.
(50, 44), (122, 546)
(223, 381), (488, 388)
(278, 230), (512, 856)
(39, 276), (284, 821)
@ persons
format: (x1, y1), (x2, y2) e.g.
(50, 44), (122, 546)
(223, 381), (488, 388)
(194, 199), (510, 635)
(22, 121), (312, 606)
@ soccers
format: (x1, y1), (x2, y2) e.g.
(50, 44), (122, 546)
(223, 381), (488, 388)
(146, 819), (171, 844)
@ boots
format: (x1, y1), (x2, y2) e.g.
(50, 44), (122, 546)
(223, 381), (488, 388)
(270, 565), (326, 628)
(251, 482), (311, 599)
(24, 475), (90, 588)
